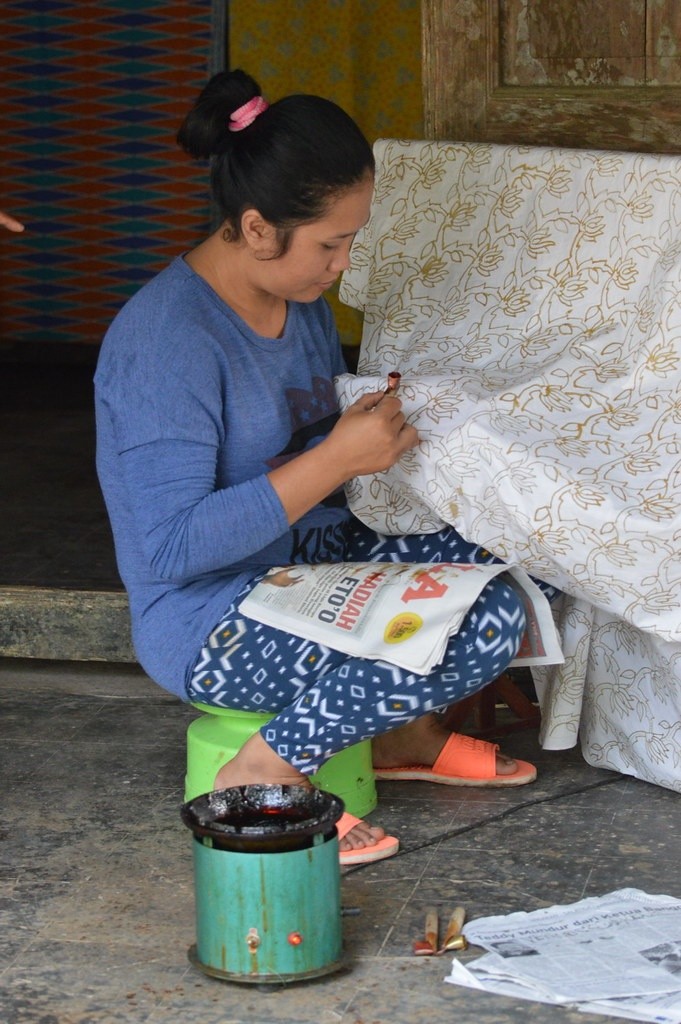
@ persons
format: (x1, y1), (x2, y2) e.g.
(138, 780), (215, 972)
(0, 211), (25, 233)
(86, 68), (538, 868)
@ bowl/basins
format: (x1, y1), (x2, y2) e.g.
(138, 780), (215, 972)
(179, 783), (346, 850)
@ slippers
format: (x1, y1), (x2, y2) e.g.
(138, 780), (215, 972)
(335, 811), (400, 864)
(374, 732), (537, 787)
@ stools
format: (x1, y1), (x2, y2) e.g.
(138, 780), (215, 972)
(185, 699), (378, 821)
